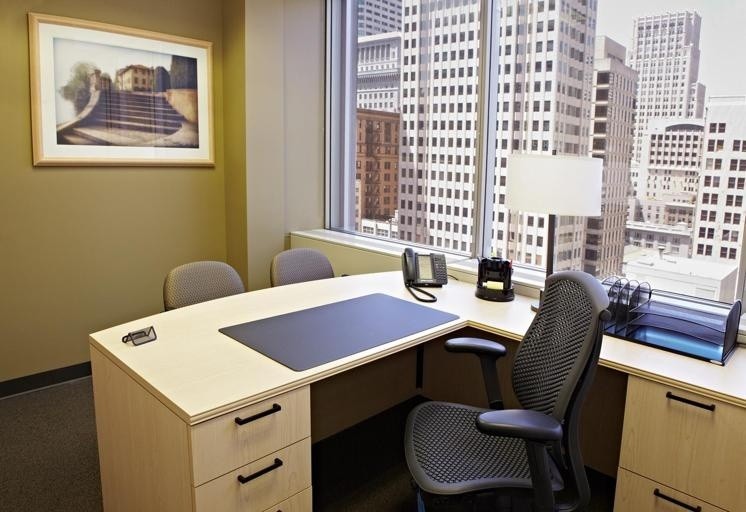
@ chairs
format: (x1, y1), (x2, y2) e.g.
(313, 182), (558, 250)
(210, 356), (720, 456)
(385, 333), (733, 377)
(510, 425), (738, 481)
(270, 247), (349, 287)
(163, 261), (245, 311)
(405, 271), (612, 511)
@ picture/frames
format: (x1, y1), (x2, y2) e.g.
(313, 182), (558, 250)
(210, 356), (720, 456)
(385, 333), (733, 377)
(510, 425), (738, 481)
(27, 12), (215, 167)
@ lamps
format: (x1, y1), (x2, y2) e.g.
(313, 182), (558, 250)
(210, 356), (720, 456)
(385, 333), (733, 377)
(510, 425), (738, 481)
(504, 154), (603, 313)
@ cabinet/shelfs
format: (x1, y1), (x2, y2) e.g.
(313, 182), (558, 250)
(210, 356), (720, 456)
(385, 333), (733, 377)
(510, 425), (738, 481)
(88, 271), (746, 512)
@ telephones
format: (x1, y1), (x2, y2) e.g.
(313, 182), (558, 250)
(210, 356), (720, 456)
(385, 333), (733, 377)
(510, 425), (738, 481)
(401, 248), (447, 285)
